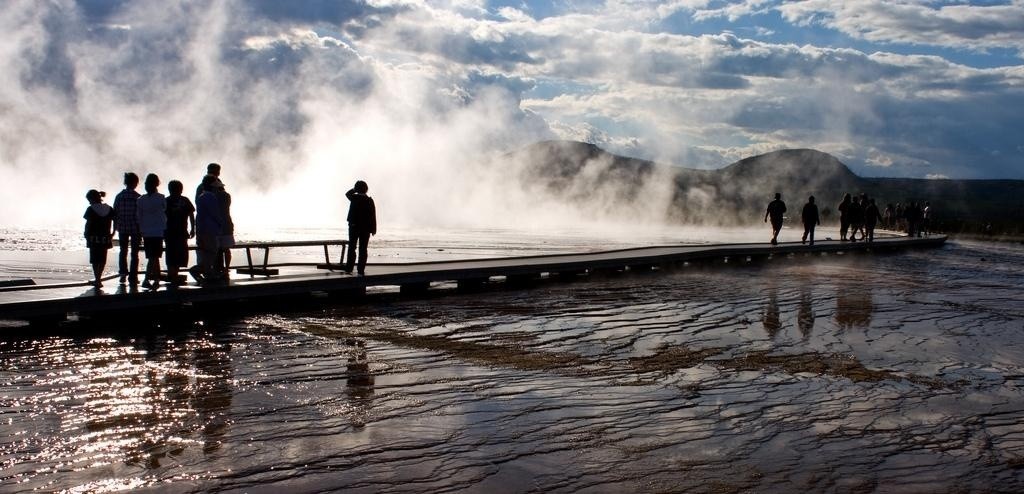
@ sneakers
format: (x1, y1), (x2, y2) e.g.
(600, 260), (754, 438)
(91, 272), (204, 291)
(769, 236), (873, 246)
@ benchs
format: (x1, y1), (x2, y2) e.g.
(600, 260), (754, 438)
(137, 239), (352, 283)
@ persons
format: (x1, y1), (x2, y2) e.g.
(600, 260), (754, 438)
(345, 180), (376, 275)
(801, 195), (820, 244)
(765, 192), (787, 245)
(83, 162), (235, 290)
(838, 191), (933, 241)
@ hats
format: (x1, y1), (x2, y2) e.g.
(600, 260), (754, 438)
(774, 193), (781, 199)
(202, 174), (225, 188)
